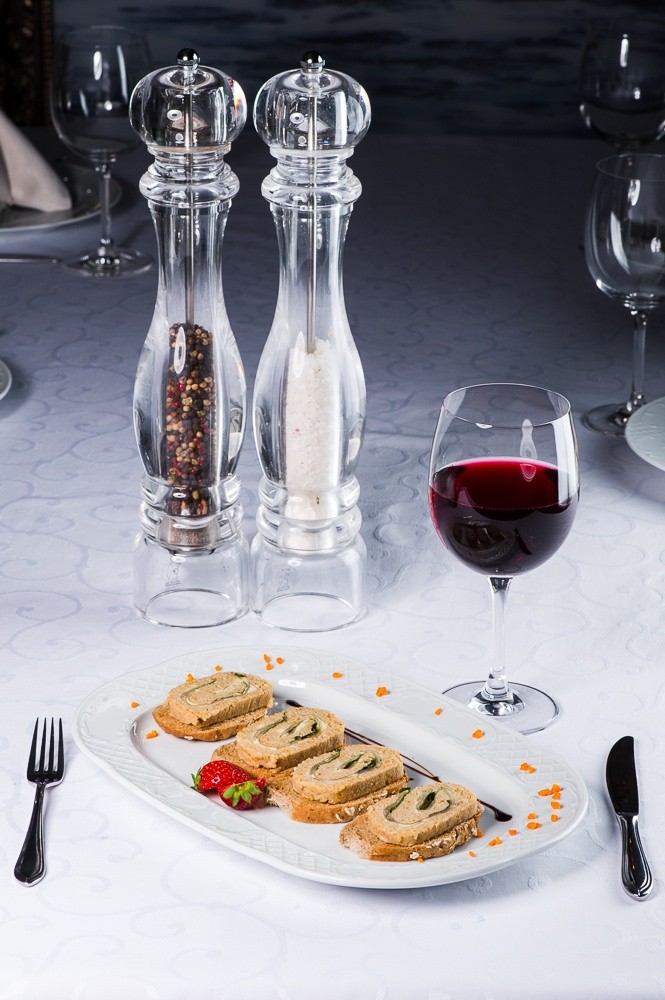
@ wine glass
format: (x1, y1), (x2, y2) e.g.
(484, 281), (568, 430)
(429, 381), (581, 734)
(51, 26), (155, 276)
(579, 18), (664, 154)
(578, 152), (665, 435)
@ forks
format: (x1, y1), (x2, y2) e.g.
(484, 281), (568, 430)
(14, 717), (65, 886)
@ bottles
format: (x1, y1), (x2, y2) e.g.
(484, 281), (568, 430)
(128, 64), (248, 557)
(251, 66), (371, 561)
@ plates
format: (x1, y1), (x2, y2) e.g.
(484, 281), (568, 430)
(625, 395), (665, 474)
(1, 159), (123, 232)
(1, 360), (13, 401)
(76, 647), (587, 889)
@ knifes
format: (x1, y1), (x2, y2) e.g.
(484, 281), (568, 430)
(606, 735), (652, 901)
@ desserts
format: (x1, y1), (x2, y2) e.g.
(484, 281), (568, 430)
(153, 671), (482, 864)
